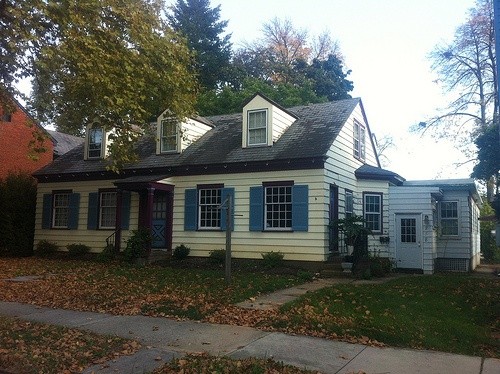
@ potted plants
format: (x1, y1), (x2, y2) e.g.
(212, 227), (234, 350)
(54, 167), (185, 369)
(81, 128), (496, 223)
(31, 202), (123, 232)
(371, 257), (391, 278)
